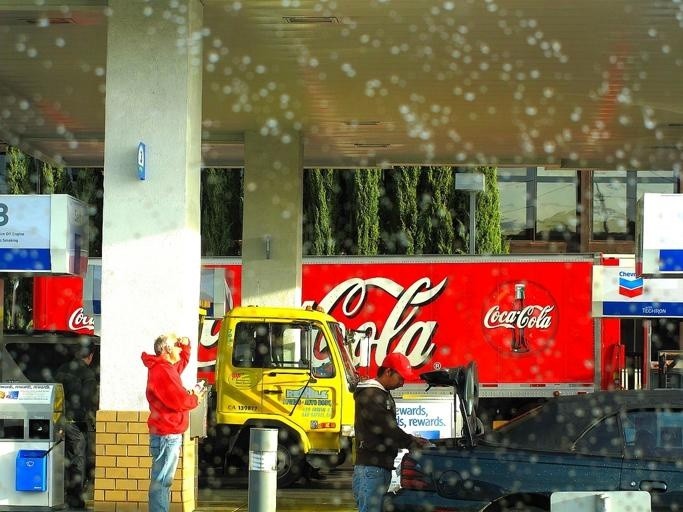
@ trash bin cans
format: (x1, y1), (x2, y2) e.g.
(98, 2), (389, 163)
(0, 383), (66, 512)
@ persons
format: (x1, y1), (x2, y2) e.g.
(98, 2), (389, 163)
(351, 352), (429, 512)
(141, 332), (207, 512)
(55, 341), (99, 512)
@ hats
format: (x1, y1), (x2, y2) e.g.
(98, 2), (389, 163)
(382, 354), (418, 381)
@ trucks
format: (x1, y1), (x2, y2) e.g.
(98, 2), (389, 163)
(0, 305), (359, 501)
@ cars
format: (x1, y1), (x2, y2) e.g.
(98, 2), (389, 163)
(392, 360), (683, 510)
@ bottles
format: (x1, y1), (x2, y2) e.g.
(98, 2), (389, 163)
(510, 283), (531, 353)
(191, 379), (205, 394)
